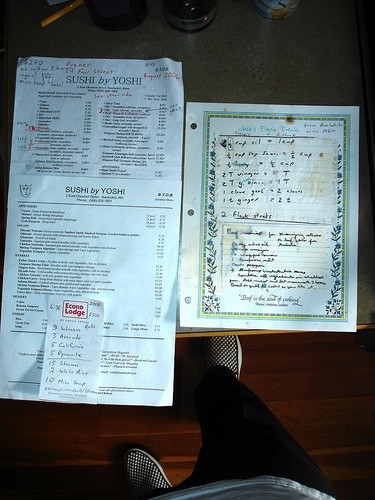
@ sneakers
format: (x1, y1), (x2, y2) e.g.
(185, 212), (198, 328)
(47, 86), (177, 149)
(122, 446), (173, 499)
(203, 335), (242, 381)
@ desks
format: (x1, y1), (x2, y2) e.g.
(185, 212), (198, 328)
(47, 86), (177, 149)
(0, 0), (375, 339)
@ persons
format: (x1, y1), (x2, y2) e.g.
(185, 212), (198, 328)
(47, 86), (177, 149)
(122, 336), (335, 499)
